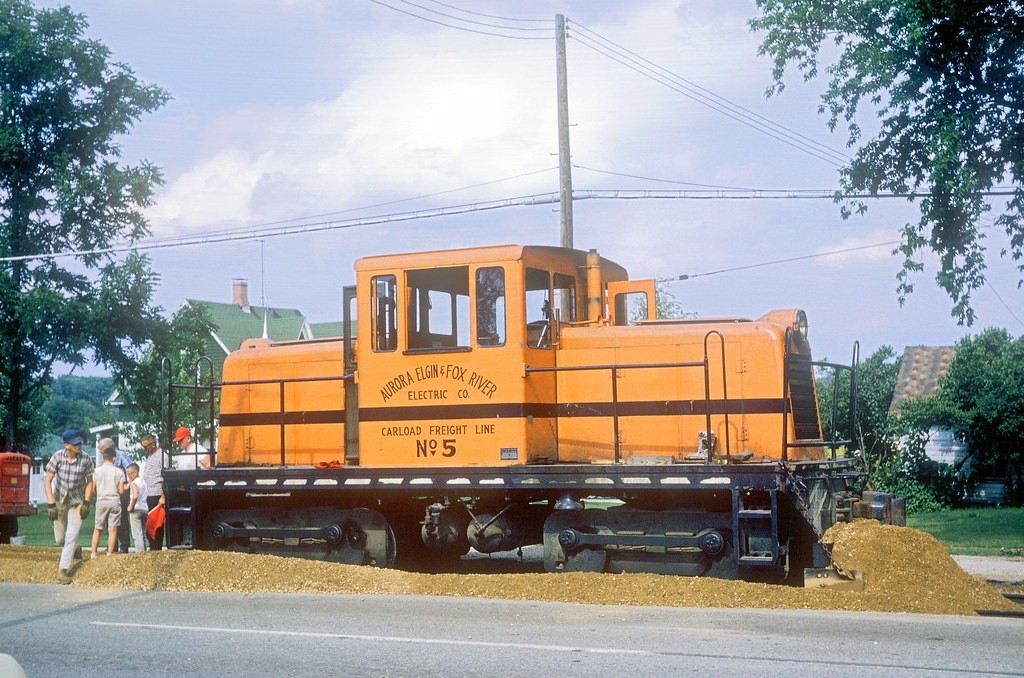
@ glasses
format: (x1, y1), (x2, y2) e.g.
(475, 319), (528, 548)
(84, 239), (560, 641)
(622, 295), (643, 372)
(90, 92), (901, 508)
(143, 443), (152, 449)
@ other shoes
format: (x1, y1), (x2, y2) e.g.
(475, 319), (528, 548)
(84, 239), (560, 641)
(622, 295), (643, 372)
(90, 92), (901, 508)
(57, 569), (71, 584)
(106, 553), (112, 556)
(91, 553), (97, 559)
(74, 544), (83, 559)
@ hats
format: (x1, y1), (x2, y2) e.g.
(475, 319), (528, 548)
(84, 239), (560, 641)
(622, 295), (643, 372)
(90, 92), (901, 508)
(173, 427), (190, 442)
(62, 429), (84, 446)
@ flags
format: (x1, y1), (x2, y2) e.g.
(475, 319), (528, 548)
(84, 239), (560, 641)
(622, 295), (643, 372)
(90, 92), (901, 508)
(146, 505), (166, 540)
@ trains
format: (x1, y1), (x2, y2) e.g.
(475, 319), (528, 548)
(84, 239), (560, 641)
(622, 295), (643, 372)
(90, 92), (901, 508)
(160, 244), (868, 592)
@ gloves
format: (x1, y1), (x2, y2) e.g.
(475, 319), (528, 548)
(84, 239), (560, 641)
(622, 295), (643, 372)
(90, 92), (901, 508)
(80, 500), (90, 520)
(47, 503), (59, 521)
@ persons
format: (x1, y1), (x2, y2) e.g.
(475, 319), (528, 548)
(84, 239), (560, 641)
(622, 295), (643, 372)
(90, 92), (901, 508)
(92, 435), (170, 558)
(45, 429), (94, 583)
(173, 427), (210, 470)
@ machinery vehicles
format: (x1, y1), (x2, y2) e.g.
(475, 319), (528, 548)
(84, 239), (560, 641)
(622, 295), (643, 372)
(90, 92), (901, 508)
(0, 431), (39, 548)
